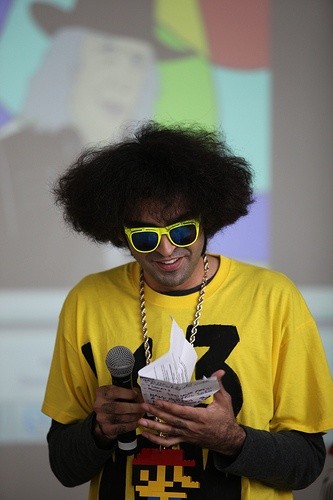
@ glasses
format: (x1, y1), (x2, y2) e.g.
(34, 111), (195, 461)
(123, 216), (203, 255)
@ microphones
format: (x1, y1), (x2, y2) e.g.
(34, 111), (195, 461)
(105, 345), (138, 455)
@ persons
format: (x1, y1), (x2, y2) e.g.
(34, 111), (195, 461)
(40, 122), (333, 500)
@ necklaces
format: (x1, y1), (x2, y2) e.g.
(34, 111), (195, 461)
(138, 254), (210, 452)
